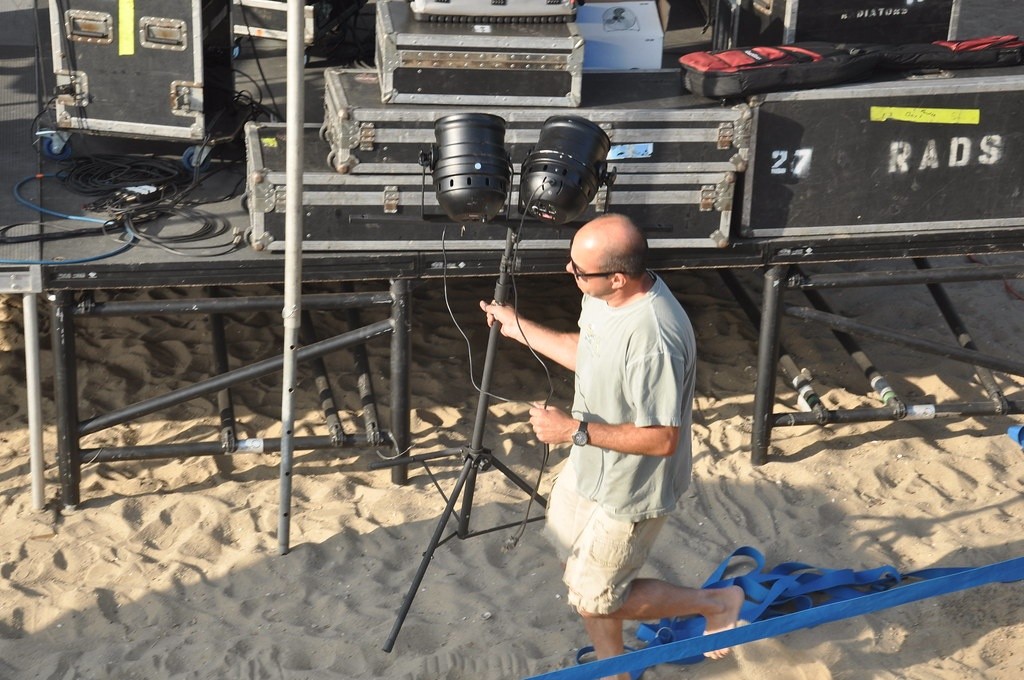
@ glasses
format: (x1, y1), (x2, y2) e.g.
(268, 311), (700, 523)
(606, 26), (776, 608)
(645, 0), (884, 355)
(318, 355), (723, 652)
(569, 255), (626, 281)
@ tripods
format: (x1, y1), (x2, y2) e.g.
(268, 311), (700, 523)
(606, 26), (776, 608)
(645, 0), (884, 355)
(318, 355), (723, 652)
(366, 227), (547, 655)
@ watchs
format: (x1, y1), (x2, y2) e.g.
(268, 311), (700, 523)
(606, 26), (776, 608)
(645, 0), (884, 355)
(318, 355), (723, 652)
(572, 421), (590, 447)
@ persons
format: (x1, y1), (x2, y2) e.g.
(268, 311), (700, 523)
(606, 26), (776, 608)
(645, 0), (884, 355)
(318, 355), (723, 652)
(478, 213), (744, 680)
